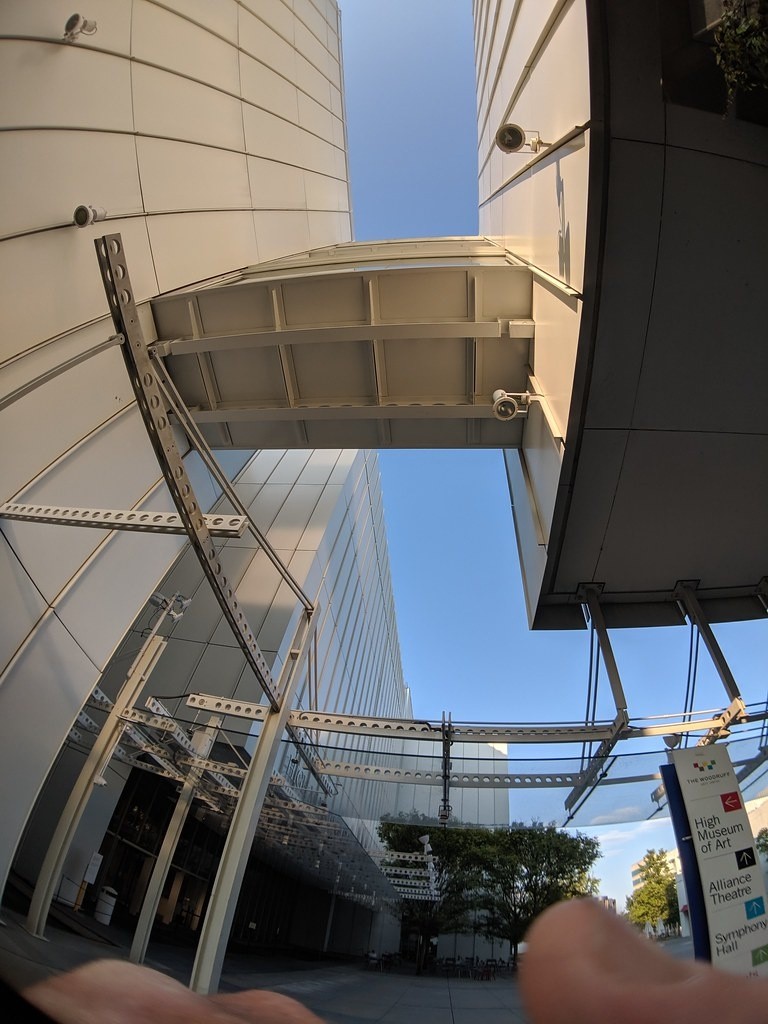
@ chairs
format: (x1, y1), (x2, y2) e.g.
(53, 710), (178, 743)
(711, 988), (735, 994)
(362, 952), (496, 983)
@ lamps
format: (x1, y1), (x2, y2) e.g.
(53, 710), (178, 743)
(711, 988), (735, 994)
(491, 389), (538, 421)
(495, 123), (552, 155)
(73, 205), (107, 228)
(63, 13), (99, 43)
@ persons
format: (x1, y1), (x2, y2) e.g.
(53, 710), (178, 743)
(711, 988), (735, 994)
(498, 958), (502, 966)
(0, 897), (768, 1024)
(365, 950), (377, 965)
(476, 959), (484, 967)
